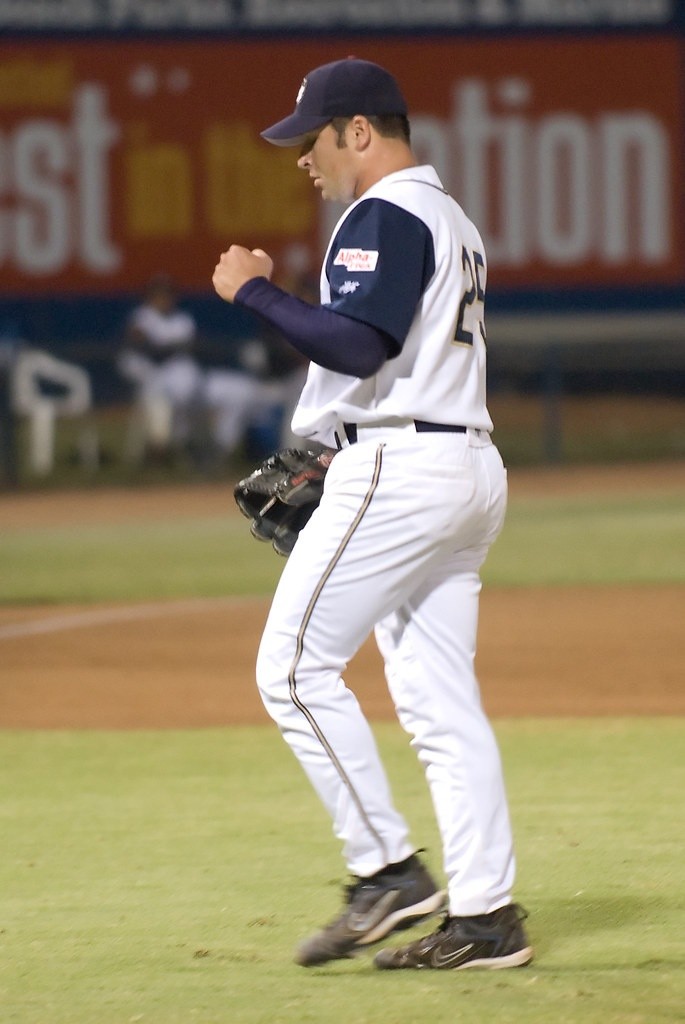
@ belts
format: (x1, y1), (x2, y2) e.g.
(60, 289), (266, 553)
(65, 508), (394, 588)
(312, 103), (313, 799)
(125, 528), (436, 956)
(333, 416), (481, 448)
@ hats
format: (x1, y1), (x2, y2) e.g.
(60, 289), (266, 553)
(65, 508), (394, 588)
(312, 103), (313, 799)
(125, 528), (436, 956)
(259, 59), (409, 146)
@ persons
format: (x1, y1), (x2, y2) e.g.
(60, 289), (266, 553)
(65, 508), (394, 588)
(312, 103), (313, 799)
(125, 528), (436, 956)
(0, 265), (316, 493)
(212, 55), (534, 971)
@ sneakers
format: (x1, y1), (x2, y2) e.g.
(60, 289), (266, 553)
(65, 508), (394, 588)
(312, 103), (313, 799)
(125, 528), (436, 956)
(290, 859), (446, 967)
(373, 906), (533, 968)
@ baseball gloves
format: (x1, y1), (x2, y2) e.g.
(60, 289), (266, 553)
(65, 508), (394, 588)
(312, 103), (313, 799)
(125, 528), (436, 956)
(231, 445), (333, 561)
